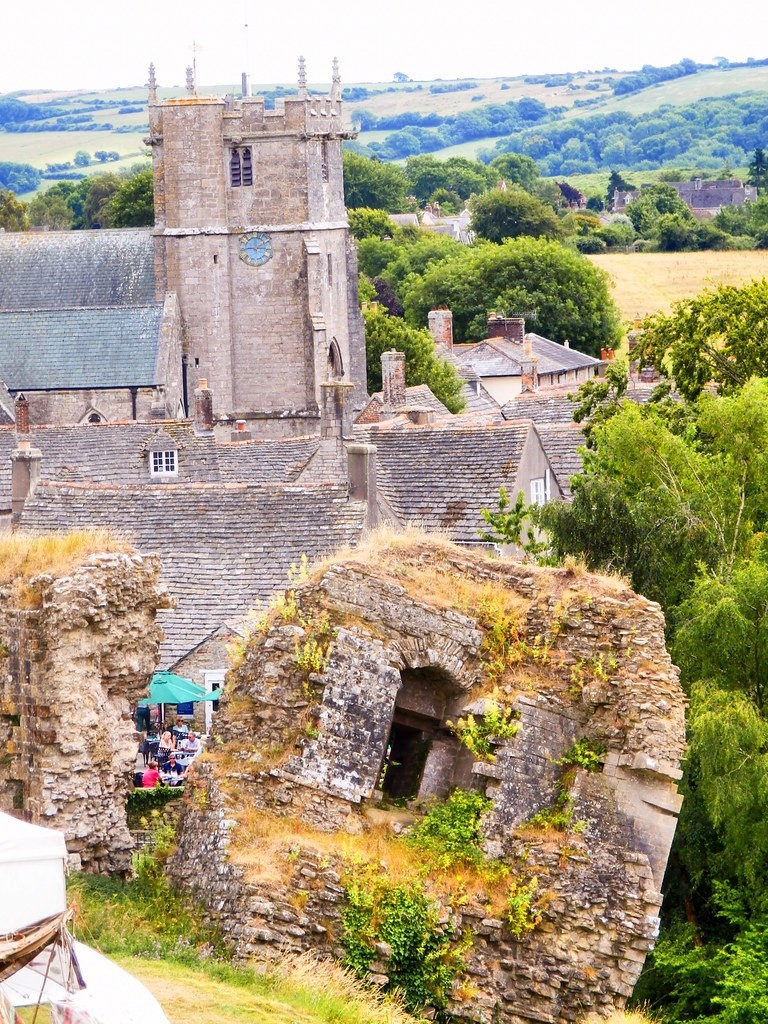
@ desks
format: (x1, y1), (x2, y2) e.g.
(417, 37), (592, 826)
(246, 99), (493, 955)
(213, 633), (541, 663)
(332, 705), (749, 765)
(140, 737), (157, 765)
(175, 751), (193, 757)
(177, 758), (192, 768)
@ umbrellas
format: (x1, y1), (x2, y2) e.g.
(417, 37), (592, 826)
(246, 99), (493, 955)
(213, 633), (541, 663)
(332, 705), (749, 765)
(139, 672), (224, 722)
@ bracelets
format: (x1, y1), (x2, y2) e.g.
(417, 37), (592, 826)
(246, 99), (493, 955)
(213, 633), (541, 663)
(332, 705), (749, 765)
(192, 748), (194, 751)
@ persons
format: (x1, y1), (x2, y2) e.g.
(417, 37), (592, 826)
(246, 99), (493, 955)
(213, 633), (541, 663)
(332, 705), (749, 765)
(136, 702), (202, 788)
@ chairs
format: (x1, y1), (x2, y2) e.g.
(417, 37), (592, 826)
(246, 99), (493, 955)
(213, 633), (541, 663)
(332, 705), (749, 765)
(134, 722), (201, 787)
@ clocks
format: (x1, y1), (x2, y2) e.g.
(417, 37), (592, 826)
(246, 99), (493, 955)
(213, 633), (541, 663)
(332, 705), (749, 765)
(239, 232), (274, 266)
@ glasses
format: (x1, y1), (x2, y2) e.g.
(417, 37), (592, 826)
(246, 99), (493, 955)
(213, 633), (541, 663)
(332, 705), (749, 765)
(170, 758), (175, 759)
(178, 720), (183, 722)
